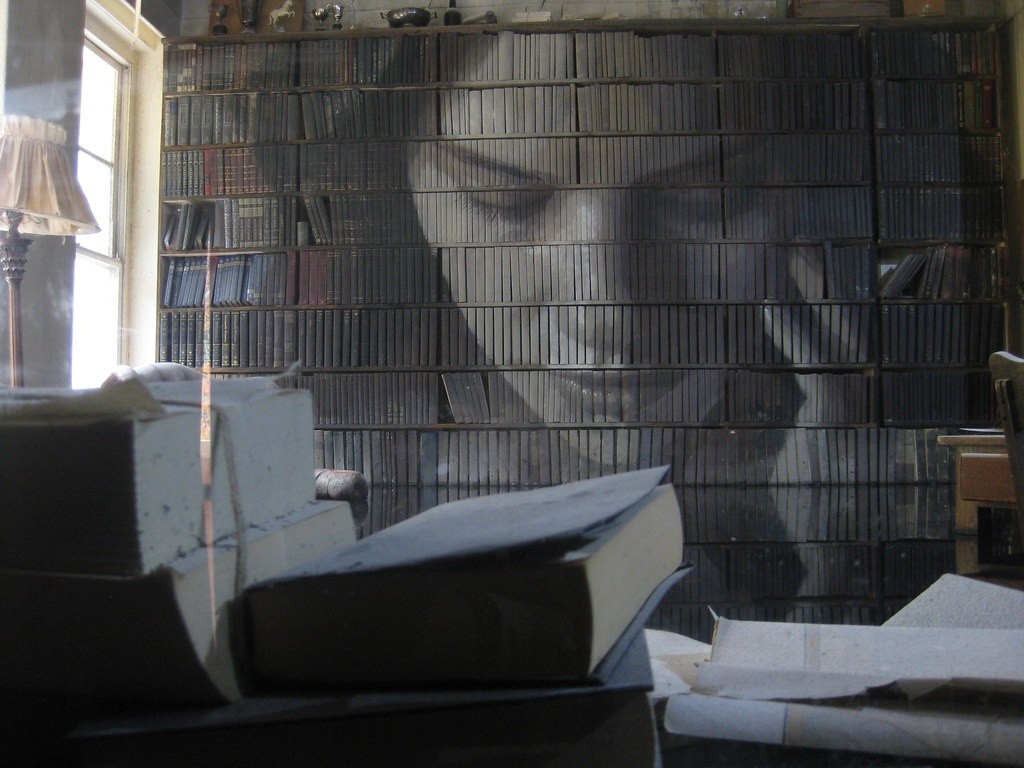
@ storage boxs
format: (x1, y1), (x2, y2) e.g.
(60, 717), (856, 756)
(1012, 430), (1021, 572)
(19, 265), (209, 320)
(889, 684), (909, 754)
(788, 0), (890, 16)
(890, 0), (961, 17)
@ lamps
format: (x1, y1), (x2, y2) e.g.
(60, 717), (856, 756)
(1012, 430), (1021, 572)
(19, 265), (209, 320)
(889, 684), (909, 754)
(0, 114), (102, 386)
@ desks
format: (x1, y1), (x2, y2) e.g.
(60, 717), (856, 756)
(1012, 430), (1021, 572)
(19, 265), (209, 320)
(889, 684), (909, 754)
(936, 435), (1016, 537)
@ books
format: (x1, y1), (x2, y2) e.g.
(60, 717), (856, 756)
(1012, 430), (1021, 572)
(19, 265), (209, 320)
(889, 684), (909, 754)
(0, 25), (1004, 710)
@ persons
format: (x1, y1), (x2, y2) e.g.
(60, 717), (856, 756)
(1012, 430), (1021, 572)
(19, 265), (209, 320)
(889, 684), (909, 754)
(262, 32), (907, 631)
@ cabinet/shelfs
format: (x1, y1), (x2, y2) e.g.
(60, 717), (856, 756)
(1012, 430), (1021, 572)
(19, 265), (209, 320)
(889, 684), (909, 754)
(157, 15), (1014, 617)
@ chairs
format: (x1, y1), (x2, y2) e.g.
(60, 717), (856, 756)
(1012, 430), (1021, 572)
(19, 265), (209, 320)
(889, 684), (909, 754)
(315, 468), (368, 541)
(988, 351), (1024, 541)
